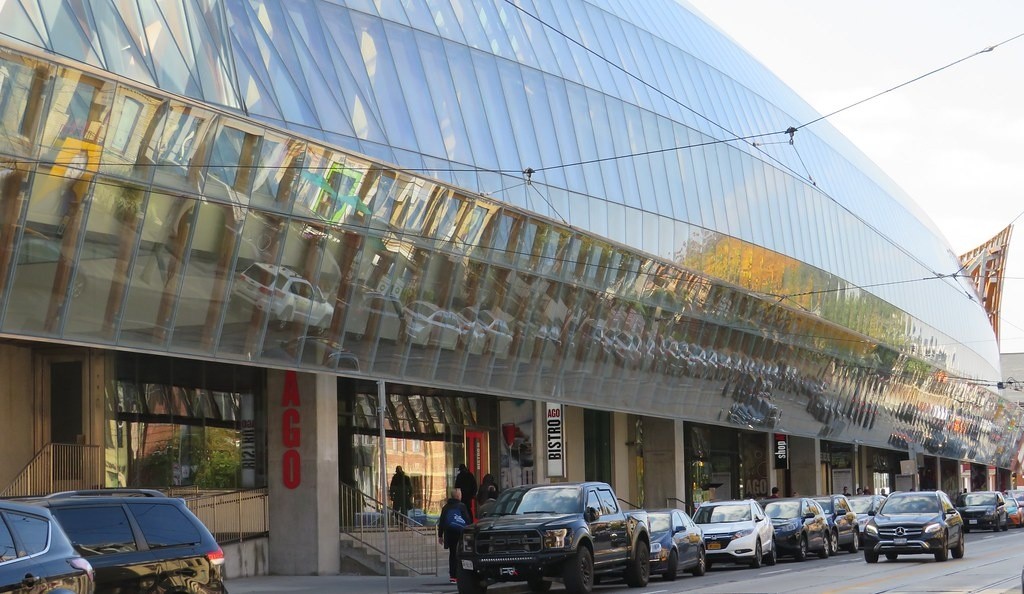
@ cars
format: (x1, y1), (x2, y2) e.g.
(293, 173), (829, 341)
(740, 497), (832, 562)
(844, 495), (887, 549)
(626, 509), (706, 581)
(1004, 489), (1024, 529)
(801, 493), (860, 556)
(459, 306), (847, 426)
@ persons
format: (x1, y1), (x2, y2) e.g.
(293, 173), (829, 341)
(477, 473), (499, 506)
(438, 488), (472, 584)
(765, 486), (779, 499)
(843, 486), (851, 496)
(956, 487), (967, 506)
(480, 485), (498, 521)
(856, 486), (914, 499)
(389, 465), (414, 530)
(792, 491), (802, 499)
(455, 465), (477, 522)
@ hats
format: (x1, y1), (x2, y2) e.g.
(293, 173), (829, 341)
(457, 464), (465, 471)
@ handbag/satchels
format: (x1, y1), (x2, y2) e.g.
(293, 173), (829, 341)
(446, 502), (467, 531)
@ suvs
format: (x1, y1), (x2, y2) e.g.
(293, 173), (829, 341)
(727, 397), (779, 429)
(684, 498), (778, 573)
(0, 500), (99, 594)
(953, 491), (1009, 533)
(328, 281), (407, 342)
(400, 300), (463, 352)
(862, 490), (965, 563)
(234, 262), (334, 335)
(22, 488), (228, 594)
(455, 482), (651, 594)
